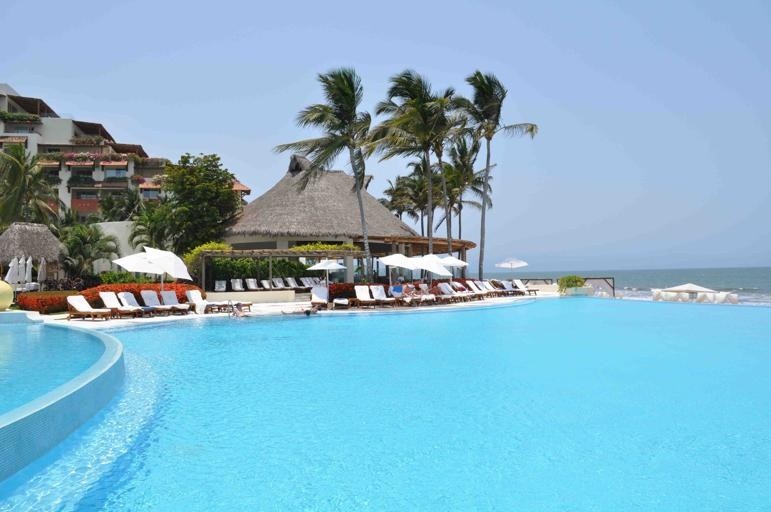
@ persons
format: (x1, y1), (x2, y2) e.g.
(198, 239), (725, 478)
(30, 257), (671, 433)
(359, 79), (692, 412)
(305, 310), (310, 316)
(232, 303), (250, 317)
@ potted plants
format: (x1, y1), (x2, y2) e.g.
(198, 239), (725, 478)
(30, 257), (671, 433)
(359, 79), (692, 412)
(554, 275), (595, 298)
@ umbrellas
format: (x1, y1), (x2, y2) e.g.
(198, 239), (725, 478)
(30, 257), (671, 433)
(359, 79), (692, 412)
(5, 256), (20, 298)
(494, 257), (528, 275)
(111, 244), (193, 305)
(18, 255), (25, 293)
(36, 256), (47, 292)
(305, 259), (347, 286)
(24, 256), (32, 292)
(378, 253), (469, 290)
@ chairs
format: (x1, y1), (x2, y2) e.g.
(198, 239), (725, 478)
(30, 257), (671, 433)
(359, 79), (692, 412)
(63, 289), (253, 320)
(308, 278), (538, 310)
(212, 276), (334, 291)
